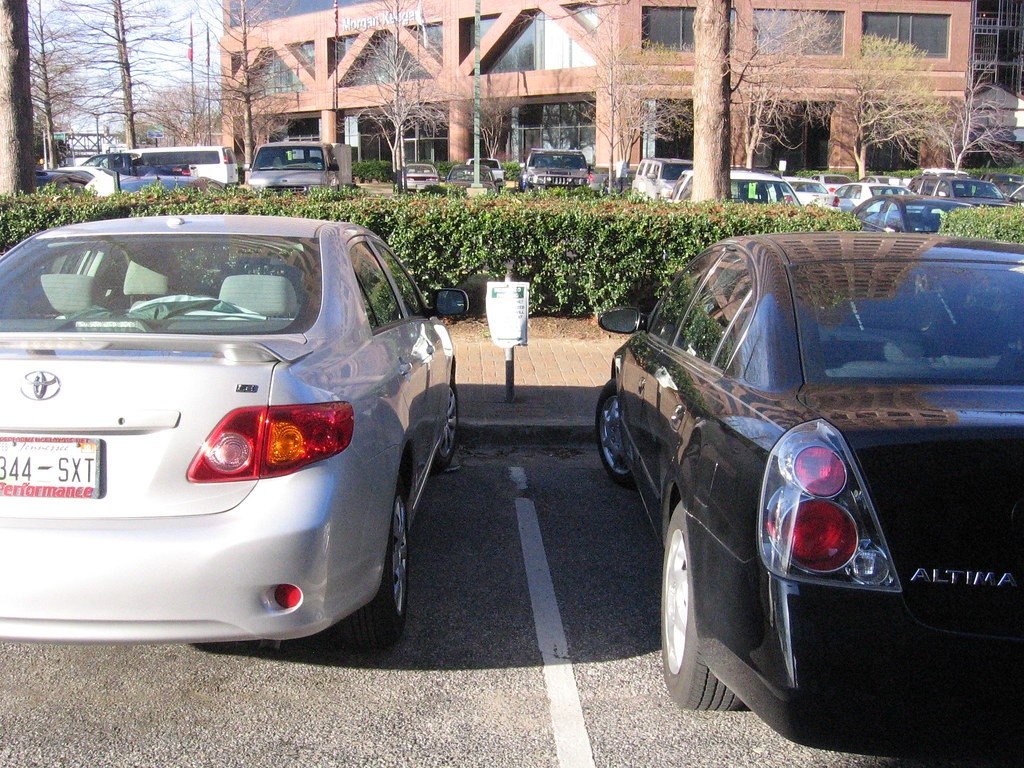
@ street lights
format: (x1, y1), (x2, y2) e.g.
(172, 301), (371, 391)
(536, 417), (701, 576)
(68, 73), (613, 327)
(92, 110), (104, 155)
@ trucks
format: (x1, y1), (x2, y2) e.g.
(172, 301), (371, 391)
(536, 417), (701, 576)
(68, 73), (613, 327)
(242, 142), (351, 195)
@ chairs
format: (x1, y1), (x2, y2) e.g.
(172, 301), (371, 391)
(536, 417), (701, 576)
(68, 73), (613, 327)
(41, 273), (98, 320)
(273, 157), (285, 167)
(218, 274), (297, 317)
(122, 261), (169, 307)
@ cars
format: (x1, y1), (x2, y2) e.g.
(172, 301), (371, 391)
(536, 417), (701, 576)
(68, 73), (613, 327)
(810, 175), (941, 215)
(589, 174), (621, 196)
(0, 215), (468, 652)
(850, 194), (975, 235)
(445, 165), (502, 194)
(755, 177), (841, 212)
(596, 232), (1024, 759)
(660, 167), (801, 208)
(36, 167), (201, 197)
(518, 151), (591, 193)
(632, 157), (694, 200)
(401, 164), (439, 190)
(908, 168), (1024, 208)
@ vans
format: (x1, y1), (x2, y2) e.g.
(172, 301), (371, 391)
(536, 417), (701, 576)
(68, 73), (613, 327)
(99, 146), (240, 188)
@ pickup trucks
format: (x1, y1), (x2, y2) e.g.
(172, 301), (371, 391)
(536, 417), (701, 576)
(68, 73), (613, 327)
(79, 152), (196, 176)
(463, 158), (505, 192)
